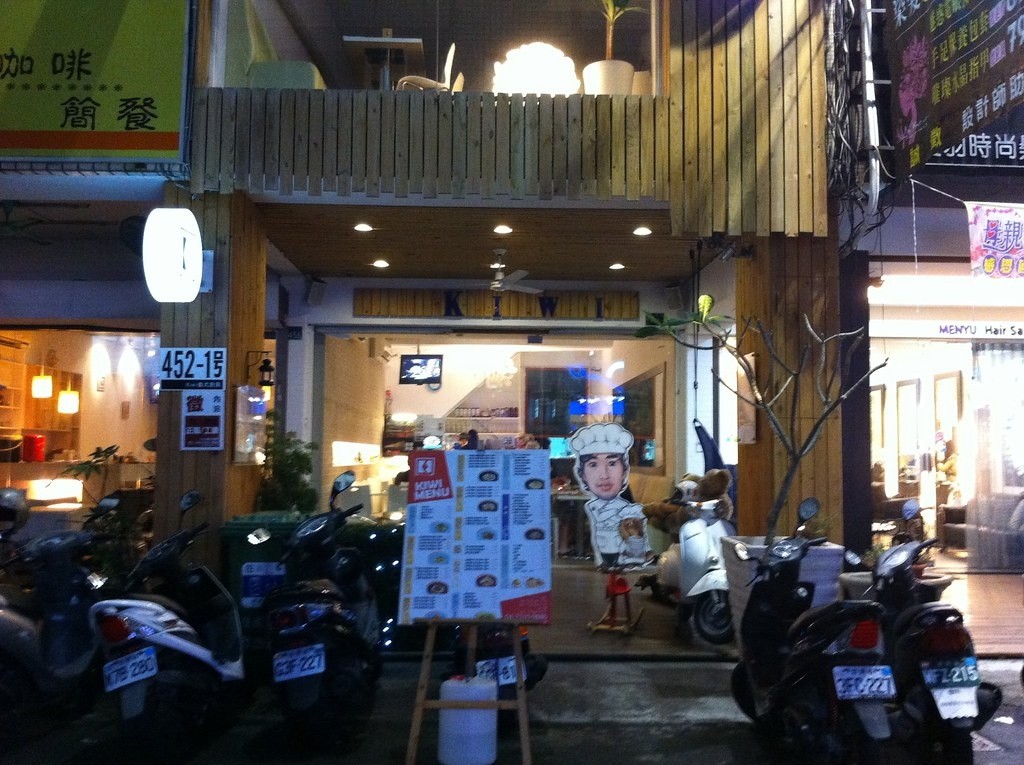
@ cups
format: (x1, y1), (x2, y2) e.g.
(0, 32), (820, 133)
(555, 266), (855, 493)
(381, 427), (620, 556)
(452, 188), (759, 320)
(63, 449), (74, 460)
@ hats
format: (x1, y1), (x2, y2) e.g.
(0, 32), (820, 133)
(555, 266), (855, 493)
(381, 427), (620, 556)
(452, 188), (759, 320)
(459, 433), (469, 440)
(412, 441), (424, 447)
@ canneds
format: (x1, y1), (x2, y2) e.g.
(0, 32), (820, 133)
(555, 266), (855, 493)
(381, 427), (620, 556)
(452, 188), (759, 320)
(455, 407), (481, 417)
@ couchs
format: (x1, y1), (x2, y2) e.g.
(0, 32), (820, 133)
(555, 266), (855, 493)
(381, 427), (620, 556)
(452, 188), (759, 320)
(938, 491), (1024, 570)
(871, 481), (924, 525)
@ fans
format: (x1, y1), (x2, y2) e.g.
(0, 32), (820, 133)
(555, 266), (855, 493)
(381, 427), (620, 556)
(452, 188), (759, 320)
(465, 247), (544, 296)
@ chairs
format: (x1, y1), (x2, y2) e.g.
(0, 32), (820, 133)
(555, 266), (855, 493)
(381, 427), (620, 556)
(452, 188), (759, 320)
(225, 0), (327, 90)
(396, 43), (456, 92)
(451, 73), (465, 93)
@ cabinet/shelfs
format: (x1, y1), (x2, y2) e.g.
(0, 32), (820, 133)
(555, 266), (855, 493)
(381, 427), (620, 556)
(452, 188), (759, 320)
(0, 360), (83, 463)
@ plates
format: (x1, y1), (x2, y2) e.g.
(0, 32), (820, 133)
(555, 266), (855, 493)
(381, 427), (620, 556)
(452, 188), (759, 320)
(475, 574), (498, 588)
(476, 528), (498, 541)
(427, 552), (450, 566)
(478, 471), (500, 482)
(426, 582), (448, 595)
(525, 528), (545, 539)
(511, 578), (546, 589)
(478, 500), (498, 512)
(525, 479), (545, 489)
(429, 521), (451, 533)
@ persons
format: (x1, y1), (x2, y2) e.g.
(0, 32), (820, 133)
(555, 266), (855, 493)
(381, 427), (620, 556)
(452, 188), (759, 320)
(450, 429), (541, 449)
(935, 439), (958, 484)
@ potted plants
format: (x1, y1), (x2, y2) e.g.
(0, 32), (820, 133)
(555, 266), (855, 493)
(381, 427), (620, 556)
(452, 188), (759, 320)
(583, 0), (652, 96)
(839, 543), (954, 610)
(910, 545), (939, 580)
(631, 290), (890, 656)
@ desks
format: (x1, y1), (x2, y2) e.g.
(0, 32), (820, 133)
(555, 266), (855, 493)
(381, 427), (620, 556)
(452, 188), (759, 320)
(342, 34), (426, 90)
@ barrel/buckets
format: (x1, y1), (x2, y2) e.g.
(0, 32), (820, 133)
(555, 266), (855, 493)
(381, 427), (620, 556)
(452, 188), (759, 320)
(0, 434), (23, 463)
(23, 434), (46, 462)
(438, 676), (496, 765)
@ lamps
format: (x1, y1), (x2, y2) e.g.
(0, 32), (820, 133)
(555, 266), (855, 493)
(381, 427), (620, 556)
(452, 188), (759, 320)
(245, 349), (275, 388)
(30, 361), (53, 400)
(57, 375), (80, 414)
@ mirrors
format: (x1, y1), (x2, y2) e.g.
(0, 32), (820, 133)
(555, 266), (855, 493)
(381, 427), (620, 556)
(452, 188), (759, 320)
(613, 361), (667, 477)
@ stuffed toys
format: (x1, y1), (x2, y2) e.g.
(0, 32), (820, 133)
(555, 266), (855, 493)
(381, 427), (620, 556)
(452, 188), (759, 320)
(640, 465), (735, 538)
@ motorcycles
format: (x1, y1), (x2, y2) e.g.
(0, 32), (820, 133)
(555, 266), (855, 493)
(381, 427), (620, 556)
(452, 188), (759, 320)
(0, 467), (1001, 765)
